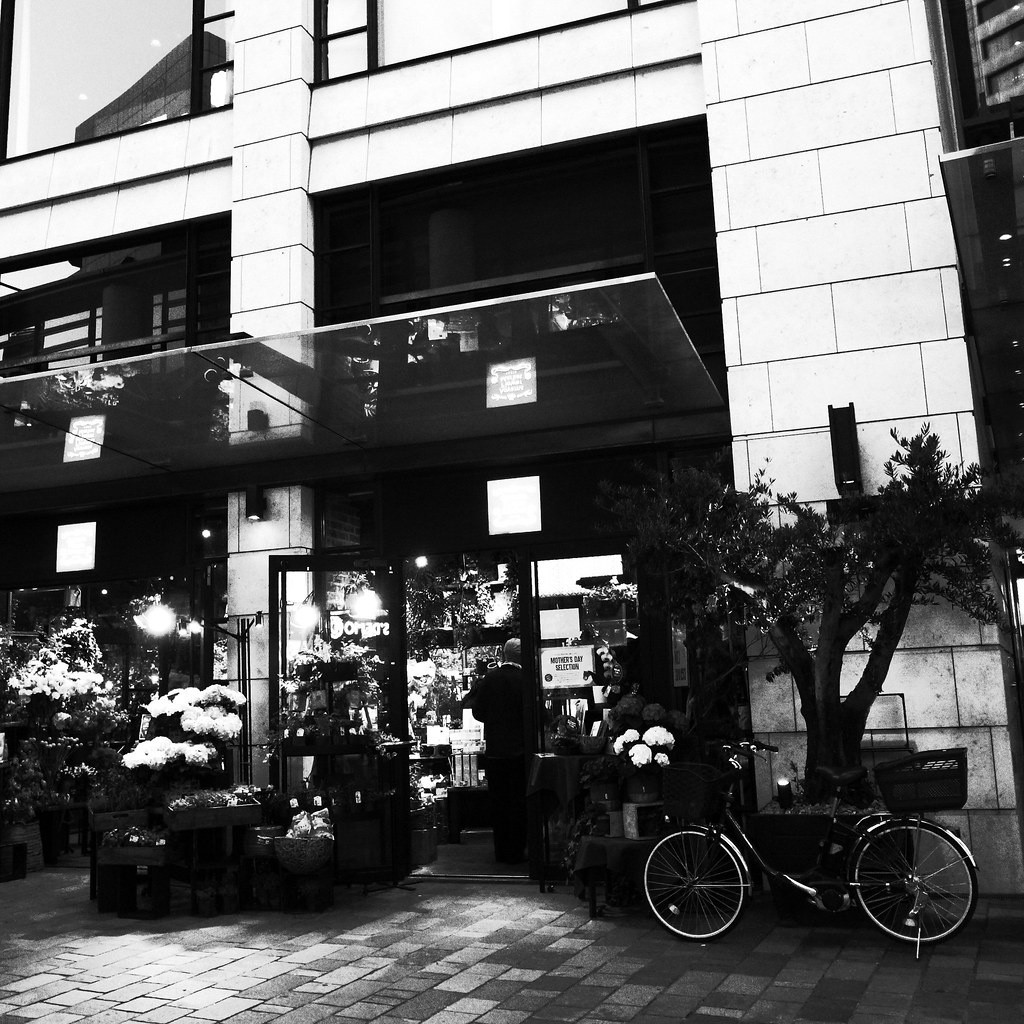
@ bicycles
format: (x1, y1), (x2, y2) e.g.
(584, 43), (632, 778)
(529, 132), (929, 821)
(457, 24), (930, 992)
(641, 737), (980, 961)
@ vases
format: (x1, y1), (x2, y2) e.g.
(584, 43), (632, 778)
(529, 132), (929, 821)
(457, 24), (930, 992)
(0, 738), (417, 917)
(628, 771), (661, 802)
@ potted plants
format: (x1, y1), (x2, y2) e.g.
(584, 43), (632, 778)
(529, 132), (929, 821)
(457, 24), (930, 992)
(588, 422), (1024, 918)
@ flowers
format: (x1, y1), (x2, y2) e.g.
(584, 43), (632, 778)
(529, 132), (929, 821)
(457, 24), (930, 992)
(612, 726), (675, 776)
(0, 594), (433, 836)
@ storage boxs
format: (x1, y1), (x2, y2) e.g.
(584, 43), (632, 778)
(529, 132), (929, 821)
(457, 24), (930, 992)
(873, 748), (968, 819)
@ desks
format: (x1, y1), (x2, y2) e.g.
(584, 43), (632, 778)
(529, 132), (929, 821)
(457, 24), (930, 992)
(530, 752), (616, 892)
(582, 835), (670, 922)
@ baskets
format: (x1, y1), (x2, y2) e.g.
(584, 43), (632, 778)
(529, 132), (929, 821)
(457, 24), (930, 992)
(661, 762), (730, 825)
(872, 747), (968, 813)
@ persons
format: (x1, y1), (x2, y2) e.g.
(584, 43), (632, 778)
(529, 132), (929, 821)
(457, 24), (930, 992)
(472, 639), (548, 865)
